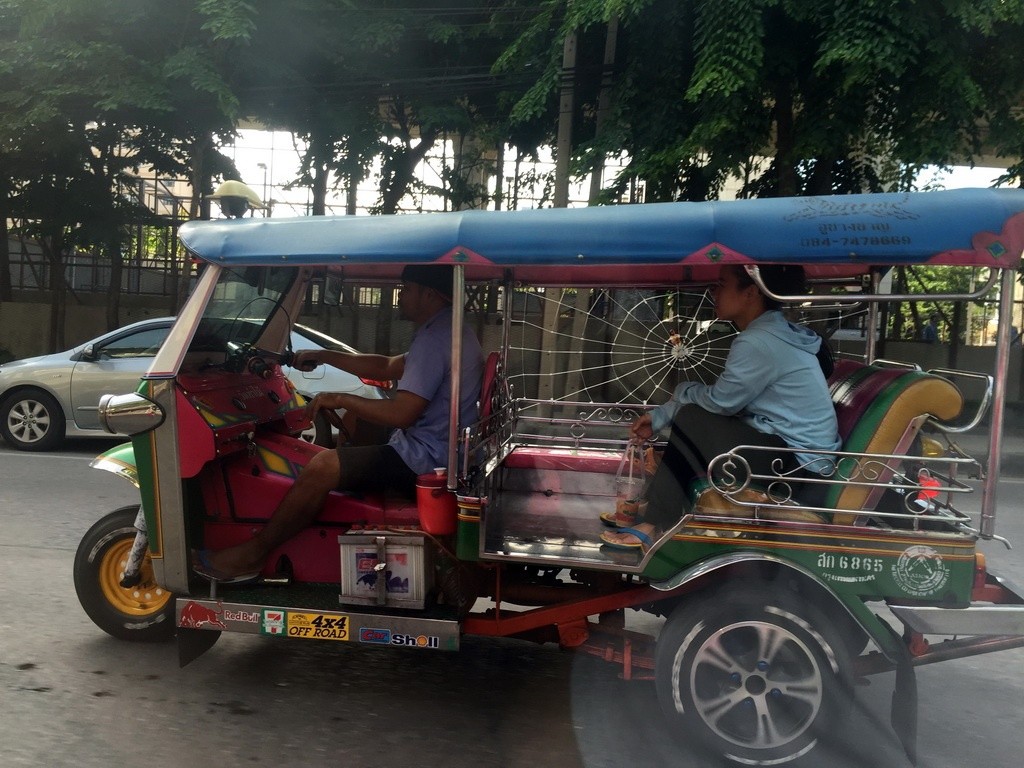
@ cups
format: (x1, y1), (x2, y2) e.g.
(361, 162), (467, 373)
(616, 477), (645, 527)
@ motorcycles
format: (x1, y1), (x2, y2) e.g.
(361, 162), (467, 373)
(73, 188), (1024, 767)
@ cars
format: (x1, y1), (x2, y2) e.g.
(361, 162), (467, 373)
(1, 317), (388, 451)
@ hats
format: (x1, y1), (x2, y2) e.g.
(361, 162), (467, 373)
(397, 263), (454, 295)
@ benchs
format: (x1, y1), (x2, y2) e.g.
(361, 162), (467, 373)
(679, 357), (964, 526)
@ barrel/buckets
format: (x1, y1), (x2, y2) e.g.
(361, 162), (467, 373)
(416, 467), (458, 535)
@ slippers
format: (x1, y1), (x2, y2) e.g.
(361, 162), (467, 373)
(199, 550), (263, 577)
(600, 504), (646, 528)
(599, 522), (652, 548)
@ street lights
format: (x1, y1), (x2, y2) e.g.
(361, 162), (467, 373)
(257, 163), (267, 218)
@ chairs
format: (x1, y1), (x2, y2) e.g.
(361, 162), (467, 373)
(371, 352), (501, 526)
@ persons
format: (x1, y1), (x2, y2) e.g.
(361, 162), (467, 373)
(600, 263), (841, 549)
(924, 315), (938, 340)
(904, 318), (926, 338)
(992, 317), (1017, 344)
(188, 264), (486, 577)
